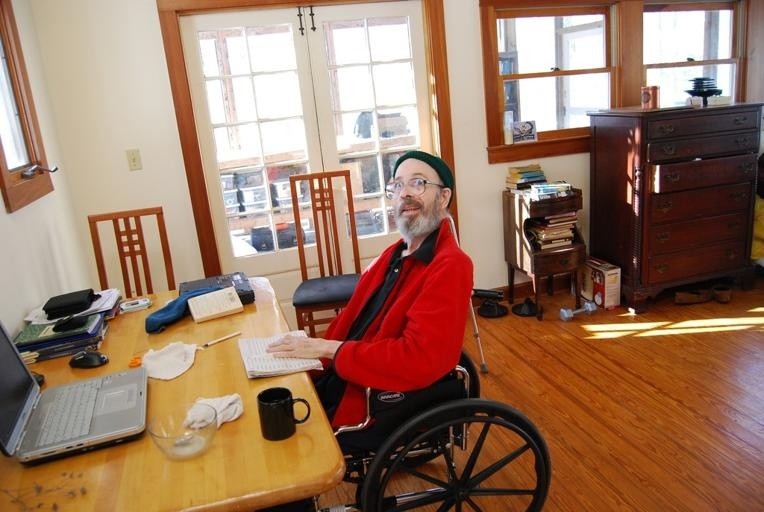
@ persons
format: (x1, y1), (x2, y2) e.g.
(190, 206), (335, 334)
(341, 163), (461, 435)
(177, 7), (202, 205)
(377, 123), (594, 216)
(266, 150), (473, 431)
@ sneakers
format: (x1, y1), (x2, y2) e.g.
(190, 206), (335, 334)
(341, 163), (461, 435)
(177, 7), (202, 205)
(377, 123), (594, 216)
(674, 285), (732, 304)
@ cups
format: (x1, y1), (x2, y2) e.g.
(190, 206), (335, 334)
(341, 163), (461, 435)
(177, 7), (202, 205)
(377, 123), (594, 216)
(256, 386), (312, 441)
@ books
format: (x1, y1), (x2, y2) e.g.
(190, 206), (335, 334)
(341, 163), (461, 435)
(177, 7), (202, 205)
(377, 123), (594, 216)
(505, 164), (578, 252)
(12, 287), (122, 365)
(179, 271), (255, 324)
(239, 330), (324, 378)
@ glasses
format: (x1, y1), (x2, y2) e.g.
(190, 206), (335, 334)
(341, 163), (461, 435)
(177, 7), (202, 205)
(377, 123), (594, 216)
(384, 178), (444, 200)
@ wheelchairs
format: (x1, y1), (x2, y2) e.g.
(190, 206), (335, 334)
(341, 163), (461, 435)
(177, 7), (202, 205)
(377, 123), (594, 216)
(321, 334), (551, 512)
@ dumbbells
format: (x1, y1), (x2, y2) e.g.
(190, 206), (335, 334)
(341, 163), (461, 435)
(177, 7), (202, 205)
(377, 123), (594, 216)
(560, 302), (597, 321)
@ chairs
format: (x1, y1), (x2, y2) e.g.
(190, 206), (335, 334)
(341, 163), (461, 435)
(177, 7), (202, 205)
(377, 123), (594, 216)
(290, 170), (365, 336)
(85, 207), (175, 300)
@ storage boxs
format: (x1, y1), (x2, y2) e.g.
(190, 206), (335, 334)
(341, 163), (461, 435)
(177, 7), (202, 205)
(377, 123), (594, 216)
(570, 256), (621, 310)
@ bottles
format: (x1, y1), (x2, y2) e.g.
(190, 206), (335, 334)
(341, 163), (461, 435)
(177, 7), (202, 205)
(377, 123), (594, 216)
(641, 85), (659, 110)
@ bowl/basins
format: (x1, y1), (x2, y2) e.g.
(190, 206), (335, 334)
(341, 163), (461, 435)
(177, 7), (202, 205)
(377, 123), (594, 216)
(147, 401), (218, 460)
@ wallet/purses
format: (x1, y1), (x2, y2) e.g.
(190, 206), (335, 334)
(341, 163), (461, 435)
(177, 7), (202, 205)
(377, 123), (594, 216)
(52, 316), (88, 331)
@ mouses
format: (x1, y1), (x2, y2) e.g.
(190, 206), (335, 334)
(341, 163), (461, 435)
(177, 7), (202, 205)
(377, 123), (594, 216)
(69, 350), (109, 369)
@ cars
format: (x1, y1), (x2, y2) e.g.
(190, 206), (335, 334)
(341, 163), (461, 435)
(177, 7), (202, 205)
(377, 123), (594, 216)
(220, 102), (414, 253)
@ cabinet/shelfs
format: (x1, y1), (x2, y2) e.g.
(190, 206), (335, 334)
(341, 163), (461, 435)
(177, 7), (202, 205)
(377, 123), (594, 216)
(502, 187), (588, 322)
(585, 103), (763, 315)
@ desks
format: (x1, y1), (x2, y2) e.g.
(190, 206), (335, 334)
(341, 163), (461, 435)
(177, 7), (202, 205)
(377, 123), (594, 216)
(0, 275), (347, 512)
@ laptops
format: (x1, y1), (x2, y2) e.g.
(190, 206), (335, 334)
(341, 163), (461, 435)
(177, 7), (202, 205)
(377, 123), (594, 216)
(0, 320), (149, 466)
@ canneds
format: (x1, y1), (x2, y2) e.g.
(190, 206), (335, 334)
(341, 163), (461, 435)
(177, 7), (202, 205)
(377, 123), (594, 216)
(640, 86), (657, 109)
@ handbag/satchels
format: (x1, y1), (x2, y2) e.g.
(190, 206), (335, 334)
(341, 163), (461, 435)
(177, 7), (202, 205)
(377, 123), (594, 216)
(42, 289), (101, 320)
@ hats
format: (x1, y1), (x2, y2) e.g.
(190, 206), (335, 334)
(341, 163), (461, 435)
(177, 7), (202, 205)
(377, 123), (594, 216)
(393, 150), (454, 208)
